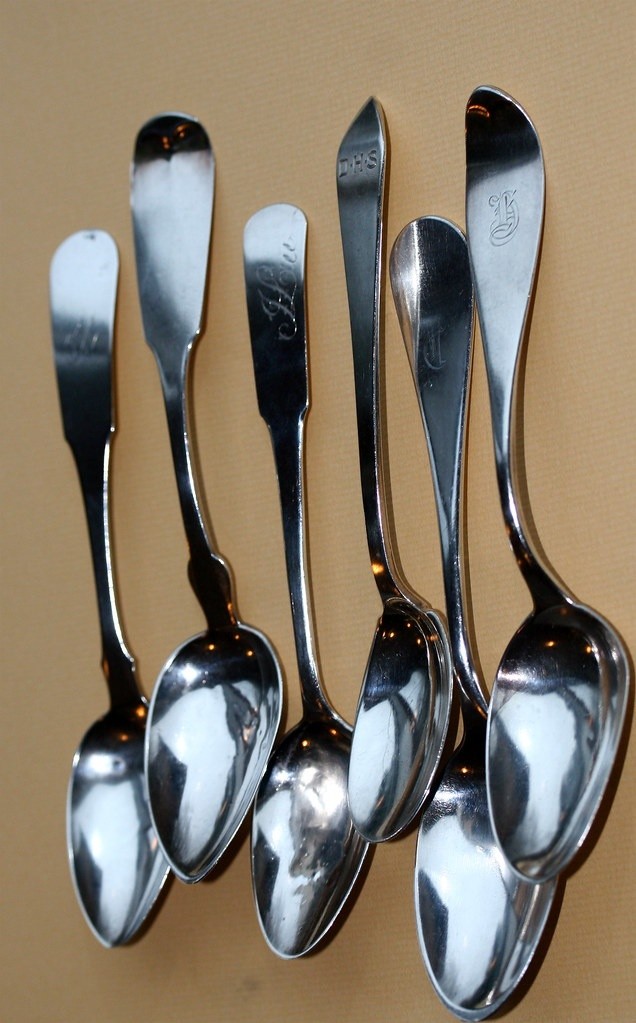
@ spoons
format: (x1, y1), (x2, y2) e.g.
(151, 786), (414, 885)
(125, 113), (287, 884)
(239, 205), (373, 959)
(48, 230), (170, 946)
(393, 213), (562, 1019)
(462, 86), (633, 890)
(333, 98), (456, 842)
